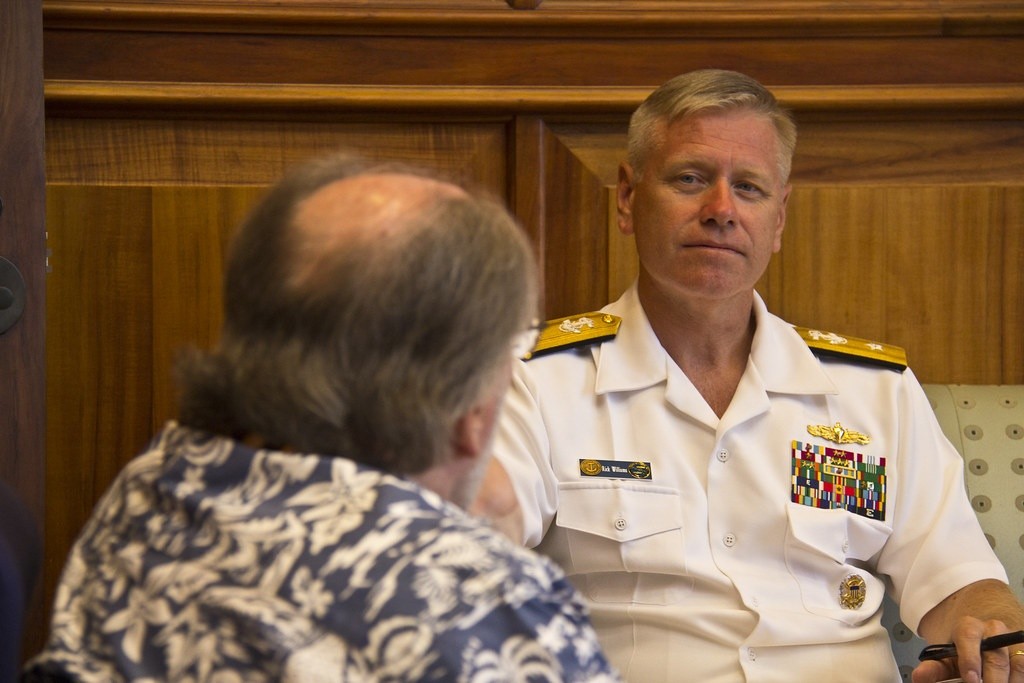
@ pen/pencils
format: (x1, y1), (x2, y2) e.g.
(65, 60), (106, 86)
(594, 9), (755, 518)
(918, 630), (1024, 661)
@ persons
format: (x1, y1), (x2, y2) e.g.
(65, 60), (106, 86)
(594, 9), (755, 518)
(16, 143), (621, 683)
(465, 67), (1024, 683)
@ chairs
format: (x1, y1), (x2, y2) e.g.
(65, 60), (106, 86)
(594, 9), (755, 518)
(872, 382), (1024, 683)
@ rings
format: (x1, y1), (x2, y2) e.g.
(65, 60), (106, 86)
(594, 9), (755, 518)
(1010, 649), (1023, 657)
